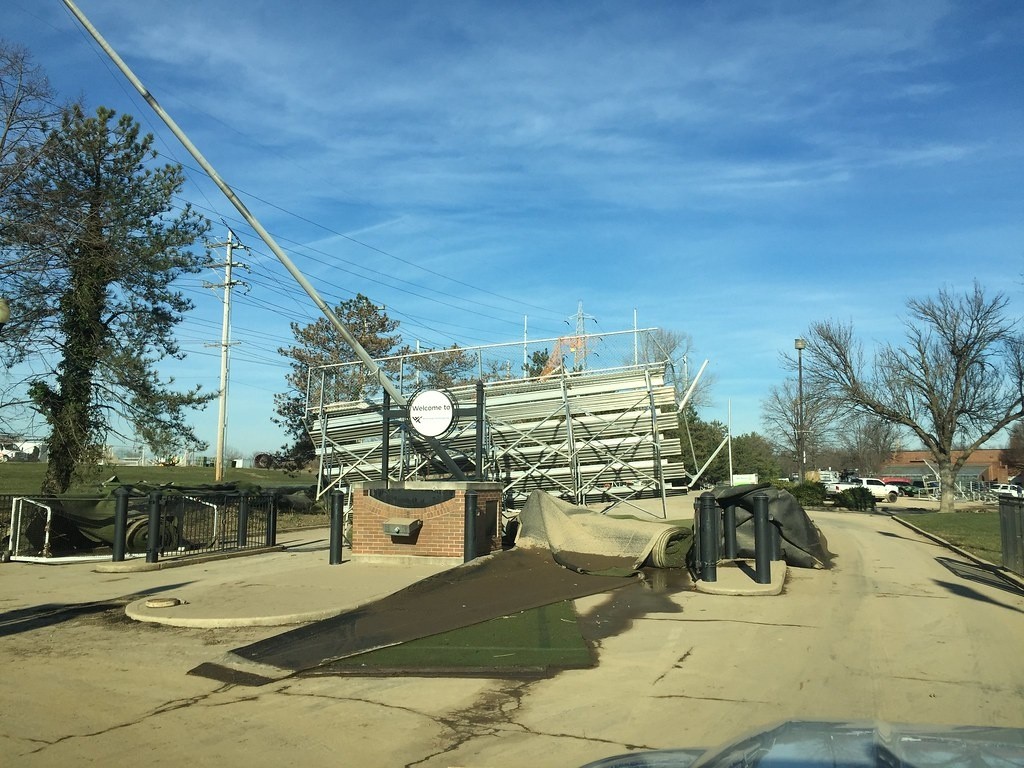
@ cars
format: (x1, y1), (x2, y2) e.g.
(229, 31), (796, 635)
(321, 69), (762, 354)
(882, 478), (913, 497)
(989, 483), (1024, 499)
(912, 479), (941, 495)
(0, 442), (27, 462)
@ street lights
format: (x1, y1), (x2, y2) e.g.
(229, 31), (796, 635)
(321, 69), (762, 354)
(794, 338), (806, 484)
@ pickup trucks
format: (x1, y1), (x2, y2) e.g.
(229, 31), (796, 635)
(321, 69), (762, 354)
(824, 477), (899, 504)
